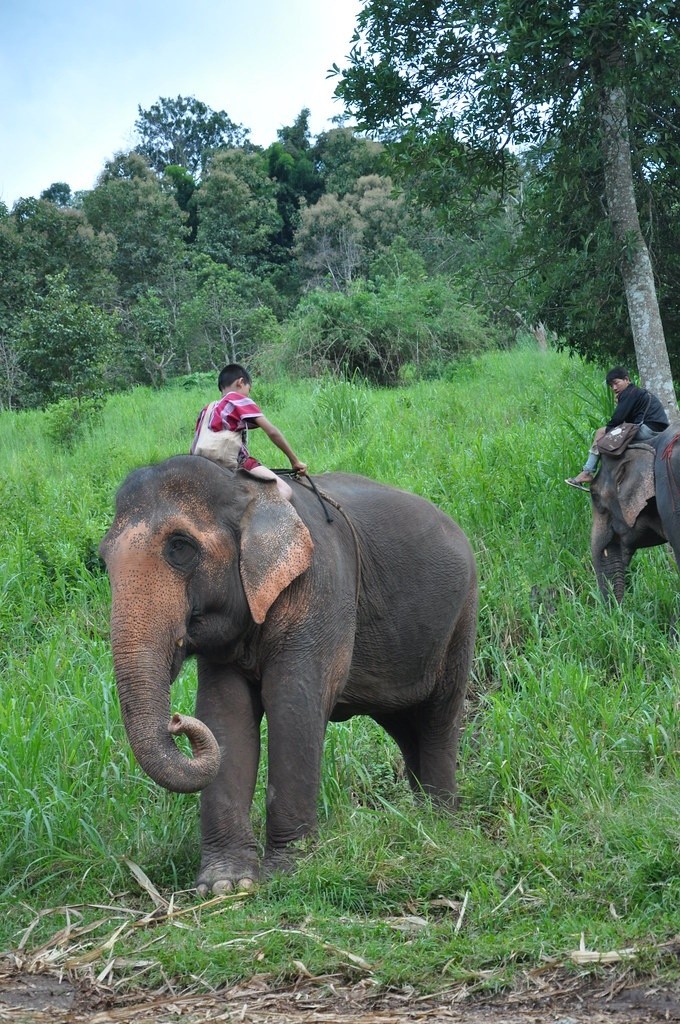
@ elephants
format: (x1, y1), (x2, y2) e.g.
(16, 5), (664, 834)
(587, 418), (680, 608)
(100, 455), (479, 901)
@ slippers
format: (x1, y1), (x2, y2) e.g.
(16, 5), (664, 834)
(564, 478), (590, 492)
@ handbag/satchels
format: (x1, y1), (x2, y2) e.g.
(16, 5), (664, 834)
(193, 401), (243, 473)
(597, 423), (641, 458)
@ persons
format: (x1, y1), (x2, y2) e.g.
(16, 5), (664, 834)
(565, 366), (669, 492)
(192, 365), (308, 502)
